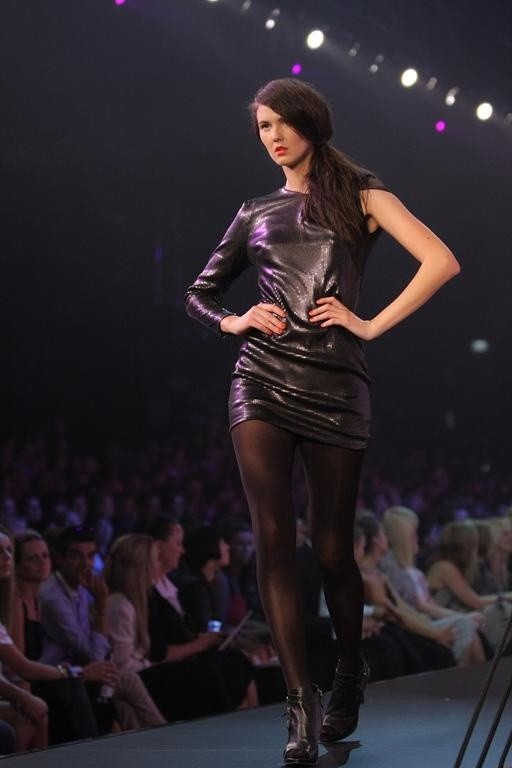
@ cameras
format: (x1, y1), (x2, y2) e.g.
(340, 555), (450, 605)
(207, 620), (221, 632)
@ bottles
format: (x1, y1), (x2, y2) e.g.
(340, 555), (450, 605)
(98, 653), (116, 704)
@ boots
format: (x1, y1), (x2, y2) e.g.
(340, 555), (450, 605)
(283, 684), (325, 766)
(321, 655), (370, 741)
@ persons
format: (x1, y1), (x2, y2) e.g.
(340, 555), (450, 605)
(181, 78), (465, 766)
